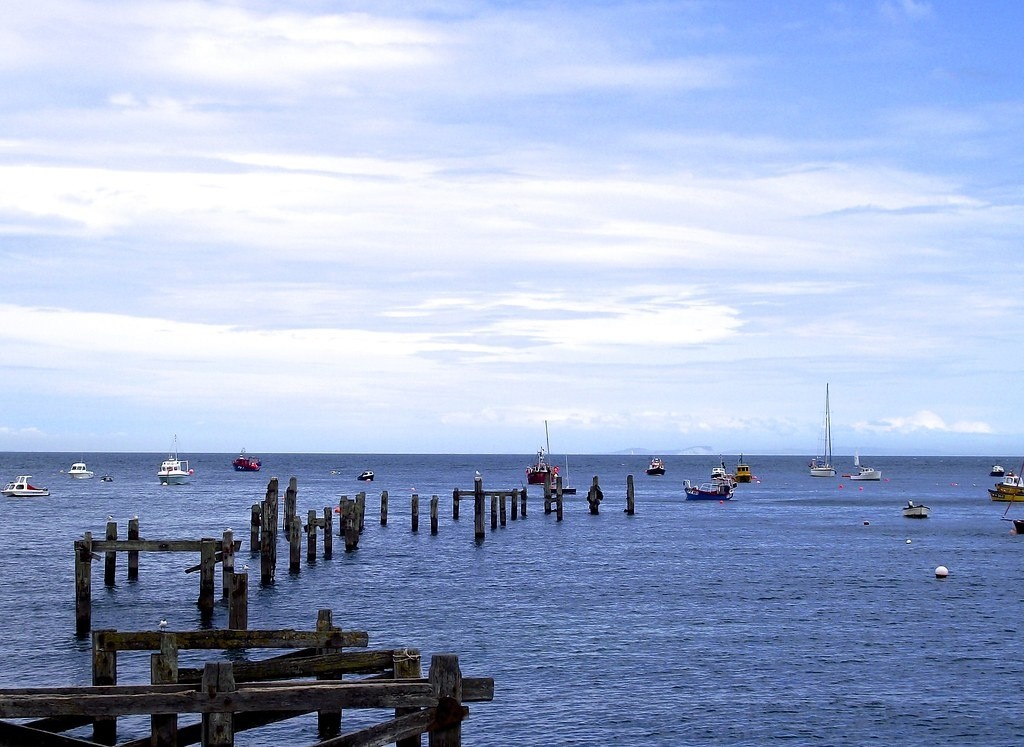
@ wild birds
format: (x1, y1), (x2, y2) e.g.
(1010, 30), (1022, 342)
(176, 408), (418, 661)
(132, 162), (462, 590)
(222, 527), (233, 533)
(133, 515), (139, 520)
(158, 619), (167, 632)
(242, 565), (250, 573)
(475, 470), (480, 477)
(107, 515), (113, 521)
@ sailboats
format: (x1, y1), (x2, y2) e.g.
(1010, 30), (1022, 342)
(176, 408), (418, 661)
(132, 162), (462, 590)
(1000, 463), (1024, 534)
(544, 454), (576, 495)
(526, 419), (559, 484)
(157, 433), (191, 485)
(810, 382), (835, 477)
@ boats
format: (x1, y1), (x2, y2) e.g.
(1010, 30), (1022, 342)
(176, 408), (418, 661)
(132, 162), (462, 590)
(357, 470), (375, 481)
(0, 476), (50, 496)
(989, 465), (1005, 477)
(987, 470), (1024, 502)
(732, 453), (752, 483)
(232, 455), (261, 471)
(850, 467), (882, 481)
(101, 476), (114, 482)
(646, 457), (667, 476)
(711, 467), (726, 480)
(902, 499), (930, 518)
(683, 454), (734, 500)
(68, 463), (94, 479)
